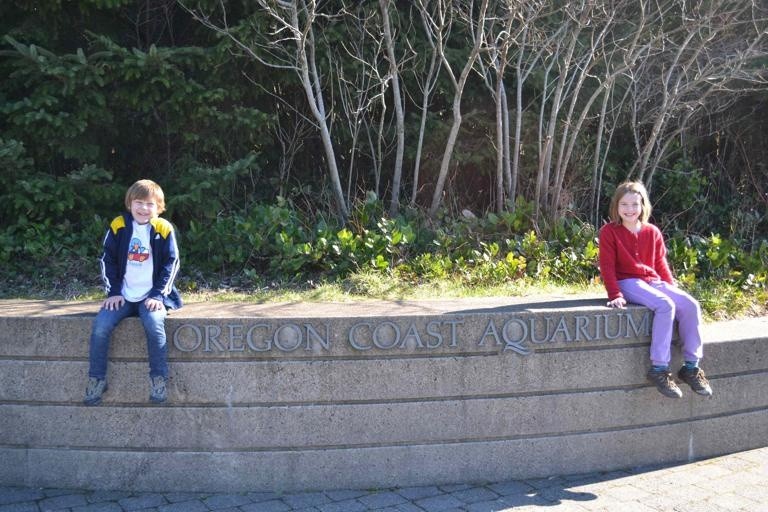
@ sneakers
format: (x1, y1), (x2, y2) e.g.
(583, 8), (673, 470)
(82, 377), (109, 408)
(677, 365), (714, 399)
(646, 366), (682, 398)
(149, 375), (170, 404)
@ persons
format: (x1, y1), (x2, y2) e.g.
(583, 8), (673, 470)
(596, 182), (713, 399)
(82, 179), (185, 407)
